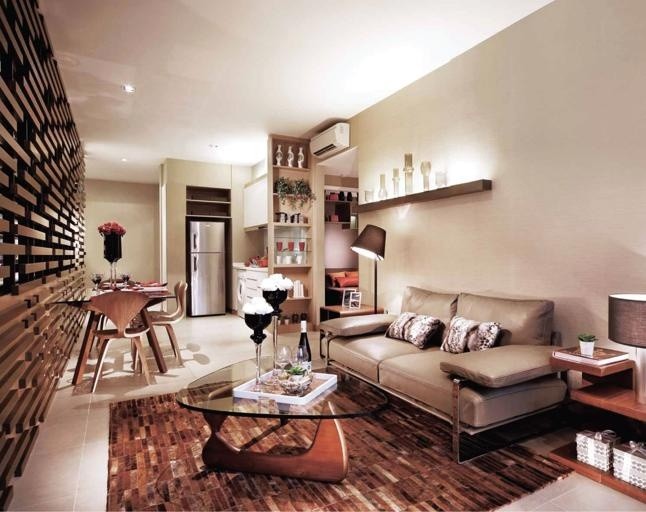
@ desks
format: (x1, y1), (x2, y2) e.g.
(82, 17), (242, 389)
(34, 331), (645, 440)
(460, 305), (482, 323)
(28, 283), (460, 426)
(54, 278), (177, 384)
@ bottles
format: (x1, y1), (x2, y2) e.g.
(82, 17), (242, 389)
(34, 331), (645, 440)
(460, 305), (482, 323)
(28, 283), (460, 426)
(297, 320), (311, 372)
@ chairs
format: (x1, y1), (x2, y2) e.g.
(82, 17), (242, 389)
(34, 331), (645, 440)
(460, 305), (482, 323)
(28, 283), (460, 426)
(89, 281), (188, 393)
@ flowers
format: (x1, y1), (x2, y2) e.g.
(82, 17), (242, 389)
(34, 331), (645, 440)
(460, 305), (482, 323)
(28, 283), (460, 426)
(97, 223), (126, 237)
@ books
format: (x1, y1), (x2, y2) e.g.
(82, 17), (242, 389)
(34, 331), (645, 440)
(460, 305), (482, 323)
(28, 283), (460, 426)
(553, 345), (629, 366)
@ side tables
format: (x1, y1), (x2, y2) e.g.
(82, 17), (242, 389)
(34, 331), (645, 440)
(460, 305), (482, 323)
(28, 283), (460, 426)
(320, 303), (383, 359)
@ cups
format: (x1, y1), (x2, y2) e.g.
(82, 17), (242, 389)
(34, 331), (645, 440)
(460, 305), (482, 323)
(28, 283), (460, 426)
(275, 239), (305, 263)
(577, 333), (594, 357)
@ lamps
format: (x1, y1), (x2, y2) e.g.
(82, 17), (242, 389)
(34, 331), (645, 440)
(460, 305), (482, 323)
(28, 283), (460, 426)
(350, 224), (386, 313)
(609, 294), (646, 404)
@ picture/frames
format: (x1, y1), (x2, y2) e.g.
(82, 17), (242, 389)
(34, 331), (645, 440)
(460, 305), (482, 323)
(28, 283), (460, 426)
(350, 292), (361, 308)
(342, 289), (355, 307)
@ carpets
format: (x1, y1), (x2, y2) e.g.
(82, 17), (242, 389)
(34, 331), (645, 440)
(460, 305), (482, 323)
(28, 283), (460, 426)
(106, 369), (573, 512)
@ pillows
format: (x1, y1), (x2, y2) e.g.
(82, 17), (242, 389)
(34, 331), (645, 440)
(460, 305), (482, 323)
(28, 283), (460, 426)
(328, 271), (359, 288)
(386, 311), (501, 355)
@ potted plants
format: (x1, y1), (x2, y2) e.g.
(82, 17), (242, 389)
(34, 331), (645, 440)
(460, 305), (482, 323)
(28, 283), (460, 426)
(287, 368), (305, 379)
(578, 334), (598, 356)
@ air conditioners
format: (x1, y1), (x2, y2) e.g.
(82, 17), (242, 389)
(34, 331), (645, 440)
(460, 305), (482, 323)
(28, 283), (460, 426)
(310, 123), (349, 159)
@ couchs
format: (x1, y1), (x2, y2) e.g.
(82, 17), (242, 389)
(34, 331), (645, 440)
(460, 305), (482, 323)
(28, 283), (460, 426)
(325, 268), (359, 305)
(320, 286), (570, 464)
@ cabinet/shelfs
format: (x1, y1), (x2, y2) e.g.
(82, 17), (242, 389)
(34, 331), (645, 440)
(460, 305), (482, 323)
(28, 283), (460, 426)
(325, 199), (351, 223)
(186, 198), (231, 218)
(549, 357), (646, 504)
(267, 134), (312, 333)
(243, 173), (267, 232)
(245, 270), (267, 301)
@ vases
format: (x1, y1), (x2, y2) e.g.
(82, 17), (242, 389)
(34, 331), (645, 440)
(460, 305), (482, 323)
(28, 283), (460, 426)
(275, 144), (304, 168)
(104, 233), (122, 287)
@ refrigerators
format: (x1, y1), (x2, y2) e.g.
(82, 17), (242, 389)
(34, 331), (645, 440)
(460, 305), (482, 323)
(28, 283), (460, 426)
(189, 220), (227, 317)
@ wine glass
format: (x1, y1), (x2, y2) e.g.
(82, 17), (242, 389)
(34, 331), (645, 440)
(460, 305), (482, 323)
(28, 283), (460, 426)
(121, 272), (129, 290)
(92, 272), (102, 296)
(275, 346), (309, 379)
